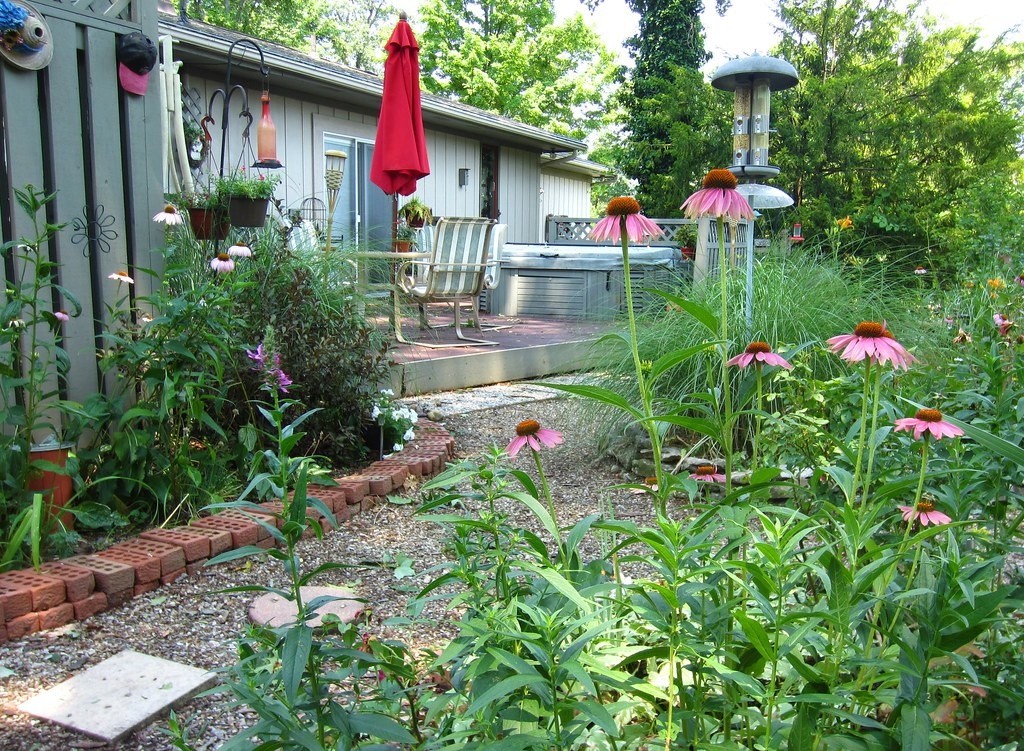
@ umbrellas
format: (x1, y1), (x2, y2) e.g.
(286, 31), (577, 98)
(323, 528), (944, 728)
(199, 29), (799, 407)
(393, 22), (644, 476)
(370, 10), (431, 336)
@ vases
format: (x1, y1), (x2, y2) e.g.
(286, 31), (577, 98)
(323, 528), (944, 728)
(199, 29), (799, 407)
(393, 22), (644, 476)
(226, 194), (270, 226)
(188, 207), (231, 240)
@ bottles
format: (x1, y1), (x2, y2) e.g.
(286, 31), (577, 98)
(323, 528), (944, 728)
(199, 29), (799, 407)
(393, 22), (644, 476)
(258, 91), (275, 160)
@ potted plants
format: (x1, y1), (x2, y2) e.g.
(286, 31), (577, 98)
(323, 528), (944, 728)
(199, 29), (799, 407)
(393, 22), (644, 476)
(391, 221), (419, 252)
(397, 195), (434, 227)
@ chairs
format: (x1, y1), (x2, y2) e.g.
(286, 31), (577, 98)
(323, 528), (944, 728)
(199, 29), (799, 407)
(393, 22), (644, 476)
(413, 225), (437, 283)
(419, 223), (513, 334)
(393, 215), (501, 348)
(277, 217), (366, 334)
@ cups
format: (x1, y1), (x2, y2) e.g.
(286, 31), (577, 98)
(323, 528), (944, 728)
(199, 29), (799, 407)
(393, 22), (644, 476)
(793, 224), (801, 237)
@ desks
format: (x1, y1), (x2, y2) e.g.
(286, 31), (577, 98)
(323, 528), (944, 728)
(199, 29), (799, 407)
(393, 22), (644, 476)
(309, 249), (435, 340)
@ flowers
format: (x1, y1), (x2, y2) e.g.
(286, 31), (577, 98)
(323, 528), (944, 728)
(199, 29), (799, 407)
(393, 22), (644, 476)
(173, 182), (237, 209)
(204, 159), (283, 204)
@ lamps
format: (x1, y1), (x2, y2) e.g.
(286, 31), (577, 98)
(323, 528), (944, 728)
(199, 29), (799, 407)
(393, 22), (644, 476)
(248, 71), (289, 169)
(324, 150), (348, 213)
(710, 51), (799, 179)
